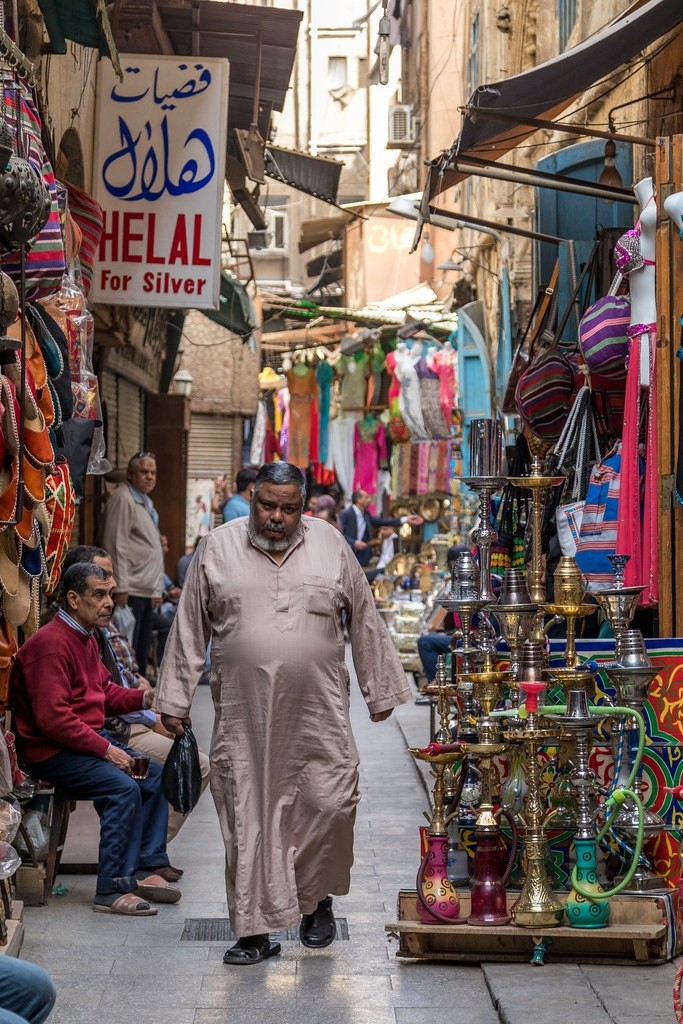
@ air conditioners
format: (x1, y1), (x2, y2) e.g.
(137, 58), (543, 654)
(387, 105), (422, 147)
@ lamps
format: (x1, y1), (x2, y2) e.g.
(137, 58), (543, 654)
(172, 348), (194, 396)
(436, 243), (495, 269)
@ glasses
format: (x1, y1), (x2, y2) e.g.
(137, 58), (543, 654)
(132, 452), (156, 459)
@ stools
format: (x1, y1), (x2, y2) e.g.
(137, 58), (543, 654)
(42, 786), (98, 905)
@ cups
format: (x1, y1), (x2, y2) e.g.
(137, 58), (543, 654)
(131, 757), (150, 779)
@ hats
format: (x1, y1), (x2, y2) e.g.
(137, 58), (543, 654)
(444, 545), (470, 565)
(310, 494), (338, 522)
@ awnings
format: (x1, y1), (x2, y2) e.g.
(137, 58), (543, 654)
(197, 274), (257, 342)
(408, 0), (683, 260)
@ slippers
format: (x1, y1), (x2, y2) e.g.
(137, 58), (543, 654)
(223, 936), (281, 963)
(135, 875), (181, 903)
(300, 898), (336, 948)
(93, 893), (158, 916)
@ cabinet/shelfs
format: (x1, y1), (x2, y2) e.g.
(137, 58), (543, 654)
(384, 742), (683, 966)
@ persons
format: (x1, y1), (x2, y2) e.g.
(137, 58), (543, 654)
(101, 450), (203, 673)
(56, 543), (209, 882)
(0, 953), (57, 1024)
(212, 462), (424, 565)
(613, 176), (683, 386)
(150, 459), (413, 966)
(414, 543), (469, 693)
(258, 341), (458, 471)
(6, 563), (182, 918)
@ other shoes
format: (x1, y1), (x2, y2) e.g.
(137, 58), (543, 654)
(152, 866), (183, 881)
(0, 82), (90, 701)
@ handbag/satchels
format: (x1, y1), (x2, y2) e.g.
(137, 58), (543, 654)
(112, 605), (136, 645)
(490, 241), (646, 597)
